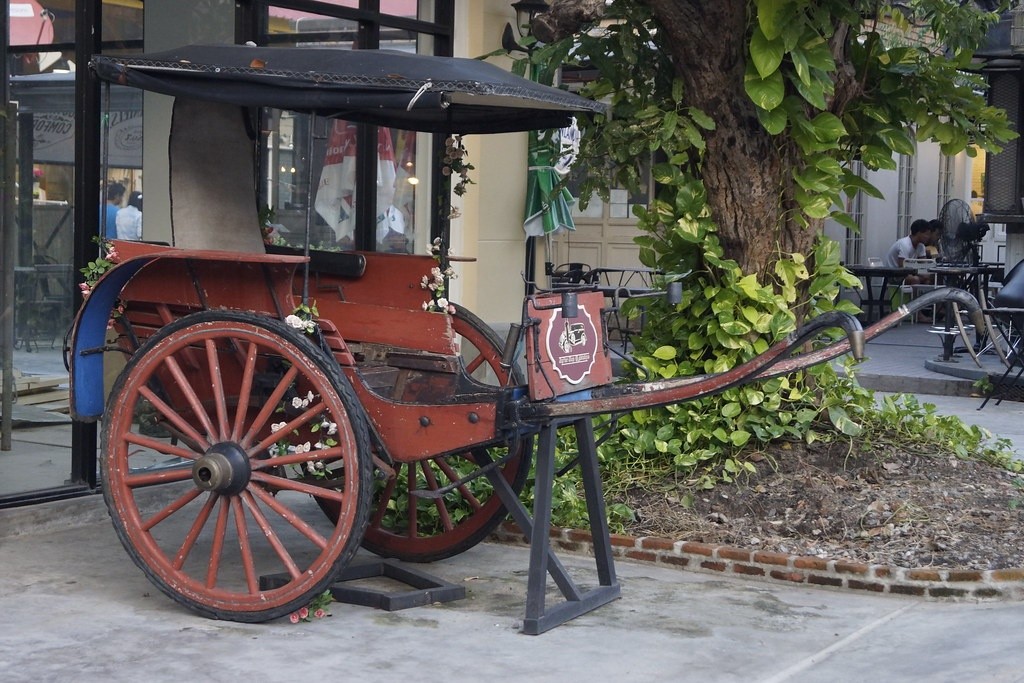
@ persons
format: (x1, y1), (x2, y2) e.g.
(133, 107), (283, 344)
(99, 183), (143, 243)
(885, 219), (944, 322)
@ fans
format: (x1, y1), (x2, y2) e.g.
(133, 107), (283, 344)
(940, 199), (1000, 354)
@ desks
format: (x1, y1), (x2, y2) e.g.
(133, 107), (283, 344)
(974, 262), (1005, 308)
(588, 267), (657, 352)
(15, 267), (58, 350)
(842, 266), (914, 324)
(977, 308), (1024, 412)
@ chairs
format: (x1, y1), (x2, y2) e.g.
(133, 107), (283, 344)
(13, 263), (73, 351)
(555, 262), (590, 286)
(867, 256), (939, 327)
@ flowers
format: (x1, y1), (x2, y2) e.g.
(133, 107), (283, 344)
(420, 201), (459, 316)
(442, 135), (477, 199)
(267, 300), (340, 478)
(79, 236), (128, 330)
(258, 200), (277, 245)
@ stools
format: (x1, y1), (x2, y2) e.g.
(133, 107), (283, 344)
(926, 265), (994, 365)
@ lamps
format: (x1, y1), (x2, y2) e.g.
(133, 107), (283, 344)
(503, 0), (551, 58)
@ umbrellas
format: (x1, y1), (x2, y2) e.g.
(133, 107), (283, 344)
(522, 43), (576, 277)
(314, 121), (405, 243)
